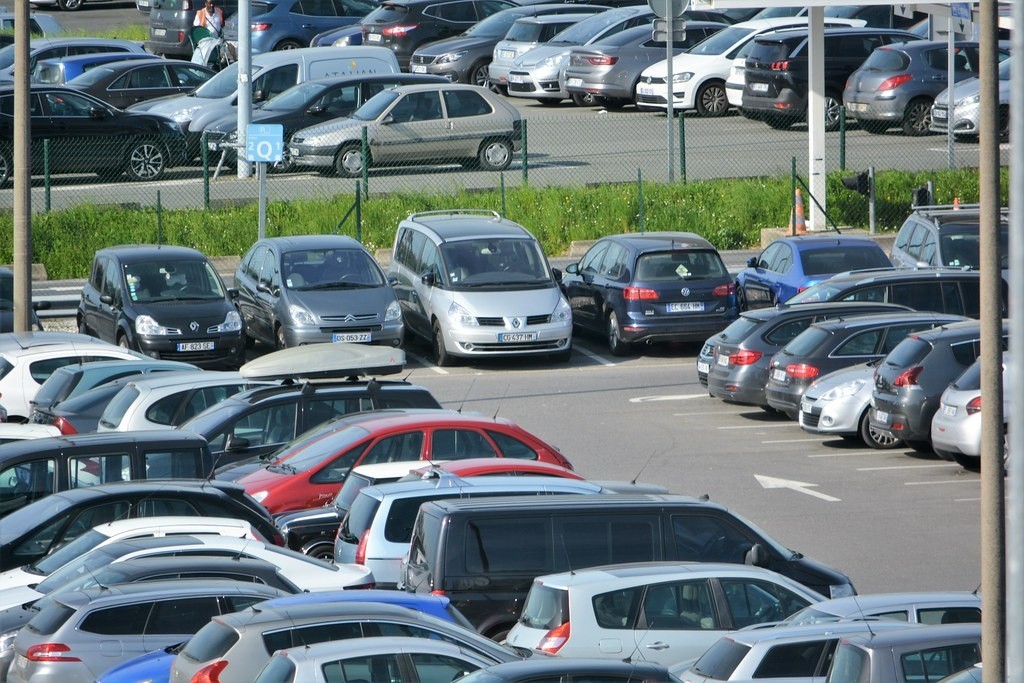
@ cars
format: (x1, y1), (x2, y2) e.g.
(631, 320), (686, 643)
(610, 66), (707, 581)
(930, 350), (1010, 476)
(564, 21), (731, 110)
(731, 235), (896, 318)
(1, 332), (985, 682)
(929, 57), (1011, 144)
(75, 243), (245, 371)
(231, 234), (405, 352)
(559, 231), (742, 358)
(0, 267), (51, 333)
(487, 13), (592, 85)
(504, 4), (737, 106)
(288, 83), (527, 177)
(0, 0), (534, 165)
(632, 17), (868, 117)
(797, 359), (903, 449)
(842, 39), (1013, 138)
(0, 86), (191, 187)
(201, 73), (456, 174)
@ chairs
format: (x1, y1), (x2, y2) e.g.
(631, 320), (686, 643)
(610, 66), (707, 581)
(267, 72), (287, 101)
(332, 89), (347, 105)
(167, 269), (190, 289)
(492, 244), (520, 268)
(416, 97), (432, 120)
(44, 471), (54, 496)
(321, 251), (348, 279)
(681, 585), (713, 629)
(265, 410), (287, 444)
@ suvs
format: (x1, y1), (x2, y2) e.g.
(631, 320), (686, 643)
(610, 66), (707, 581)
(766, 312), (979, 413)
(872, 316), (1009, 452)
(696, 266), (1009, 387)
(706, 301), (922, 414)
(890, 204), (1010, 286)
(740, 26), (924, 133)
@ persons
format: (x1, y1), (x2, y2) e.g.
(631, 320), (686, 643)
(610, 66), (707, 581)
(193, 0), (223, 37)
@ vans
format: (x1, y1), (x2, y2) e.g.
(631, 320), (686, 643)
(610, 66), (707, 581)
(393, 209), (575, 369)
